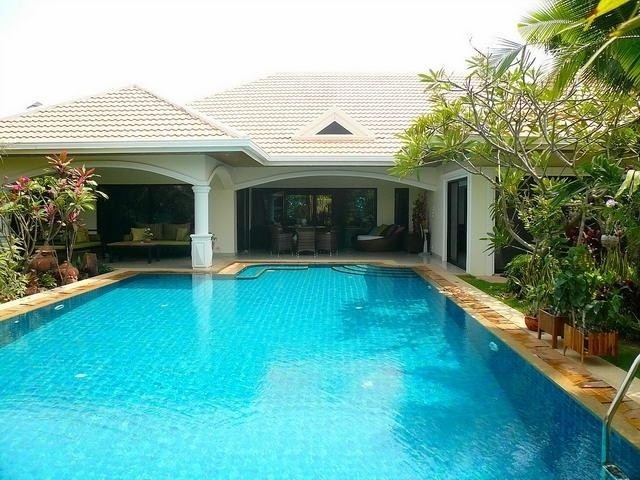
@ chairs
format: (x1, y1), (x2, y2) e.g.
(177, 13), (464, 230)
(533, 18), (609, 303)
(351, 224), (405, 252)
(270, 222), (338, 257)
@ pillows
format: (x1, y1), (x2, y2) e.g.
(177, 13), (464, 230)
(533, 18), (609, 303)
(164, 223), (191, 240)
(130, 227), (147, 241)
(176, 228), (188, 240)
(145, 223), (163, 240)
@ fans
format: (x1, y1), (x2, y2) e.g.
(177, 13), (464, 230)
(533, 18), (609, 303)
(416, 223), (431, 257)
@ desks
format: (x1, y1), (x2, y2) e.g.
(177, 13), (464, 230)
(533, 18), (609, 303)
(34, 241), (100, 267)
(106, 241), (190, 265)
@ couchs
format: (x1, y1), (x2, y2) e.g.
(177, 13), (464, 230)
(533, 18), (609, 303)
(122, 222), (192, 261)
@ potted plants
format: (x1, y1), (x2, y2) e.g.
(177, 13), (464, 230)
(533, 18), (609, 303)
(537, 246), (605, 348)
(563, 297), (618, 364)
(524, 285), (549, 331)
